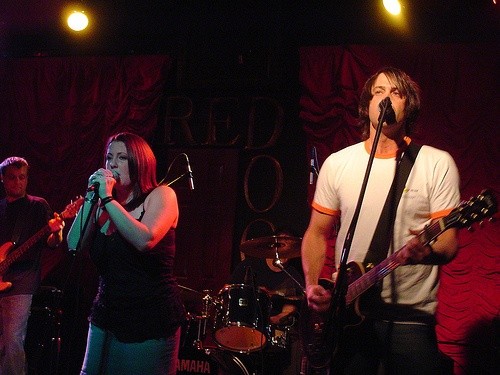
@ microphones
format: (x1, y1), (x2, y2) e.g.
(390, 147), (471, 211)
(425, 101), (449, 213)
(89, 171), (118, 191)
(377, 97), (390, 112)
(310, 151), (315, 185)
(186, 162), (195, 192)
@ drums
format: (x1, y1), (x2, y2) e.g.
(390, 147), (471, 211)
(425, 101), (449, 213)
(259, 325), (290, 354)
(212, 283), (268, 354)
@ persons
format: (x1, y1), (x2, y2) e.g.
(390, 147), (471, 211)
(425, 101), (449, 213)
(0, 156), (65, 375)
(232, 227), (304, 296)
(300, 67), (460, 375)
(67, 132), (185, 375)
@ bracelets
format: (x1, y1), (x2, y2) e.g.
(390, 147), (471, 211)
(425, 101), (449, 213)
(86, 197), (95, 204)
(102, 196), (115, 204)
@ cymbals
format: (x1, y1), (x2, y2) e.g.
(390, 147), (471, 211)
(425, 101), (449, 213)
(178, 285), (209, 312)
(239, 235), (302, 260)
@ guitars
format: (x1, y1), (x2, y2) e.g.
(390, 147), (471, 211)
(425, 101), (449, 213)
(1, 194), (83, 292)
(297, 187), (500, 369)
